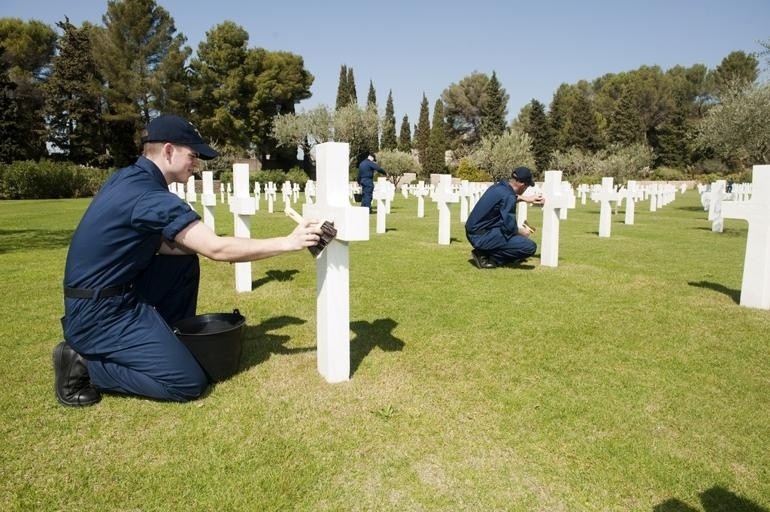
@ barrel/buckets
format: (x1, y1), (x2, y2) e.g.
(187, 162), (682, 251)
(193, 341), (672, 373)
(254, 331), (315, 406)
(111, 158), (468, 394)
(354, 194), (362, 202)
(170, 309), (245, 382)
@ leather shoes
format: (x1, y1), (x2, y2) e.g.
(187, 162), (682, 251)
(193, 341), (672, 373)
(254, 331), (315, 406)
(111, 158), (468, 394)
(472, 249), (496, 269)
(53, 342), (102, 407)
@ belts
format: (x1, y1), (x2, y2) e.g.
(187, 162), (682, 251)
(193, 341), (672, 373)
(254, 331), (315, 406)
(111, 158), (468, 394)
(64, 284), (134, 298)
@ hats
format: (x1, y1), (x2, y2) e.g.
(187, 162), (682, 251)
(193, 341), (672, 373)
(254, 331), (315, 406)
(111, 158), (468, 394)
(511, 167), (535, 186)
(368, 154), (377, 162)
(139, 114), (220, 160)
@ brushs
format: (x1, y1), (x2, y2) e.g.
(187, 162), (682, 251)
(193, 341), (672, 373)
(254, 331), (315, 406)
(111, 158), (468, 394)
(522, 220), (536, 234)
(284, 206), (338, 259)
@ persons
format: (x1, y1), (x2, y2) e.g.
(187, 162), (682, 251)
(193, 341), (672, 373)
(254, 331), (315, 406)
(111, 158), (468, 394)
(49, 115), (324, 409)
(464, 165), (544, 270)
(356, 152), (390, 215)
